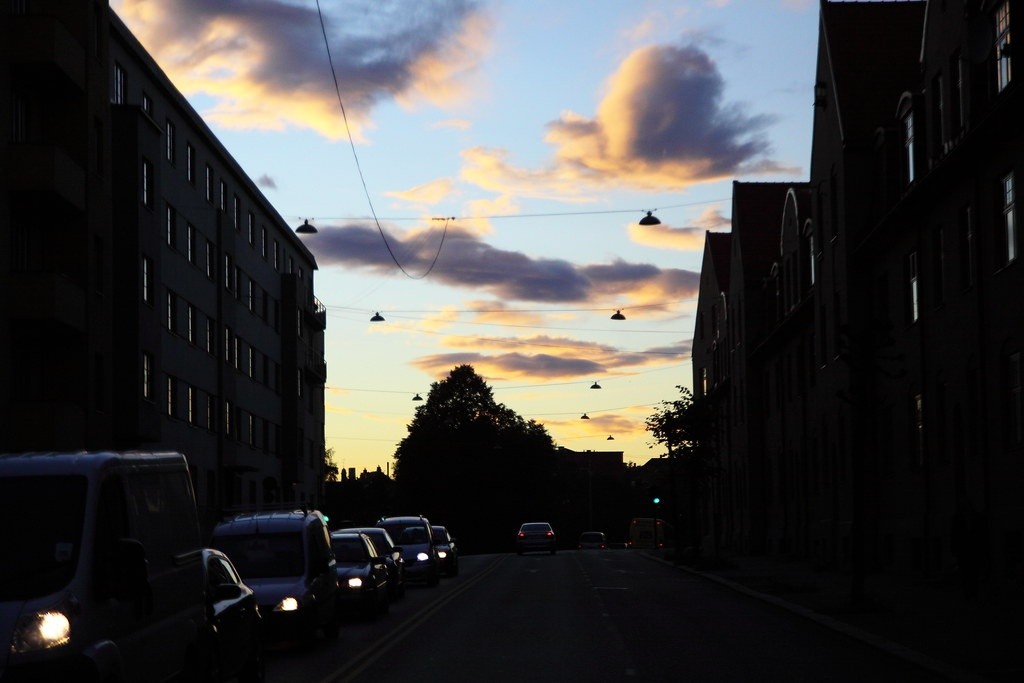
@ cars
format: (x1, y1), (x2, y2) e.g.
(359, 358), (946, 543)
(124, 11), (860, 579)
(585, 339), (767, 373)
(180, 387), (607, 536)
(517, 522), (556, 555)
(201, 546), (267, 683)
(342, 527), (406, 600)
(327, 534), (395, 620)
(431, 522), (460, 577)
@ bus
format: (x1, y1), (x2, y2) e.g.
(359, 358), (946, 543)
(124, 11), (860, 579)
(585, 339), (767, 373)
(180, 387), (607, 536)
(628, 517), (674, 552)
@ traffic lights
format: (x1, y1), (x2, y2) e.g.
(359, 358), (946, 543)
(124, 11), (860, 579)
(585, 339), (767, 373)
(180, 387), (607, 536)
(652, 496), (666, 505)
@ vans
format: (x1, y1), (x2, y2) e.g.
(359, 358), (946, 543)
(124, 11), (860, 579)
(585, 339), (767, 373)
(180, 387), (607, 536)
(207, 507), (343, 646)
(0, 448), (216, 683)
(576, 531), (608, 551)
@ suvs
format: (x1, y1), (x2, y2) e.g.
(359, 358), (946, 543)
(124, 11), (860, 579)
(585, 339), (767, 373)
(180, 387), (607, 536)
(374, 514), (440, 586)
